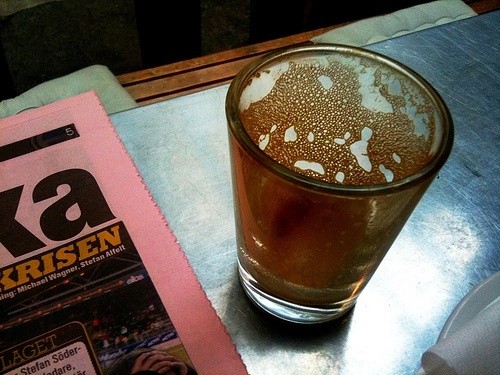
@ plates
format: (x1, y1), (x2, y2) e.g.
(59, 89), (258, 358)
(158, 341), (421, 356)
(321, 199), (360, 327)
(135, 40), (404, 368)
(431, 267), (500, 350)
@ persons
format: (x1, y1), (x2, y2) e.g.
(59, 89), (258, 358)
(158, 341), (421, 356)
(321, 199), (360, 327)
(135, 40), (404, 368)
(134, 0), (315, 70)
(108, 348), (197, 375)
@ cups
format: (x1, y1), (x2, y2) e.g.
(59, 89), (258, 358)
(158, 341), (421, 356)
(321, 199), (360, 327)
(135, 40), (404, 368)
(225, 42), (455, 326)
